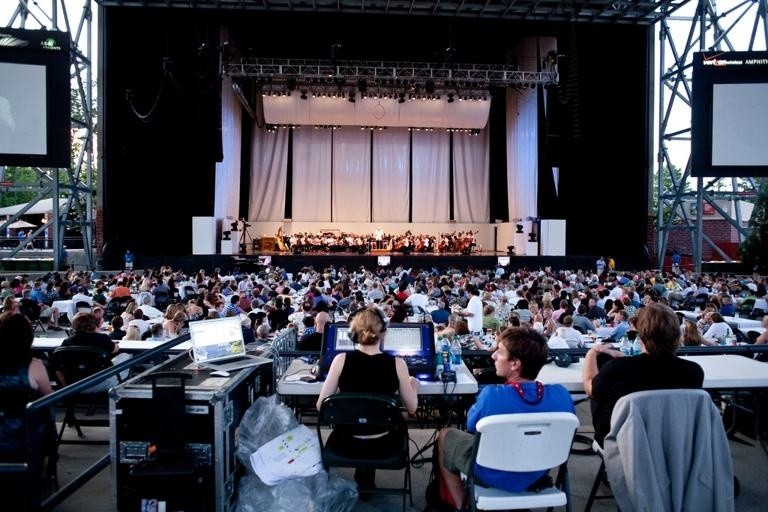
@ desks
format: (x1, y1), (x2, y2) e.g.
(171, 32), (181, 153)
(275, 354), (479, 428)
(535, 354), (766, 429)
(31, 337), (194, 355)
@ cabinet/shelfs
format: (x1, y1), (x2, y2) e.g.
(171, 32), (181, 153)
(527, 241), (538, 256)
(221, 240), (231, 255)
(230, 230), (241, 254)
(513, 232), (527, 256)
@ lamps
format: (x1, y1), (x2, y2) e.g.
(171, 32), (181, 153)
(261, 89), (487, 103)
(265, 123), (480, 136)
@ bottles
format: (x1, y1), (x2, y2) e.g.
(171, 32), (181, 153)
(442, 334), (451, 373)
(632, 332), (643, 356)
(594, 316), (607, 328)
(450, 336), (462, 372)
(435, 342), (444, 374)
(619, 333), (631, 356)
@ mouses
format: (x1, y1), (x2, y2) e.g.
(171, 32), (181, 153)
(208, 370), (230, 377)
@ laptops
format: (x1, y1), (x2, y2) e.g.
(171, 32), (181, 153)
(190, 316), (273, 372)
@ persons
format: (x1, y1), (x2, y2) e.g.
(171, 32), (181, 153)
(316, 308), (420, 503)
(0, 310), (59, 479)
(288, 231), (479, 254)
(438, 327), (576, 512)
(1, 246), (768, 359)
(583, 303), (704, 450)
(62, 311), (133, 394)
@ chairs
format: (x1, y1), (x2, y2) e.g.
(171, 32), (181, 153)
(473, 272), (766, 349)
(0, 381), (59, 512)
(458, 411), (582, 509)
(49, 346), (125, 455)
(18, 285), (212, 340)
(585, 388), (712, 510)
(317, 391), (415, 511)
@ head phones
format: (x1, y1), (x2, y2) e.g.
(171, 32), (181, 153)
(554, 352), (575, 368)
(348, 308), (388, 343)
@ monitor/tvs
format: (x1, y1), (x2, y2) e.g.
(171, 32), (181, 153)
(319, 323), (435, 377)
